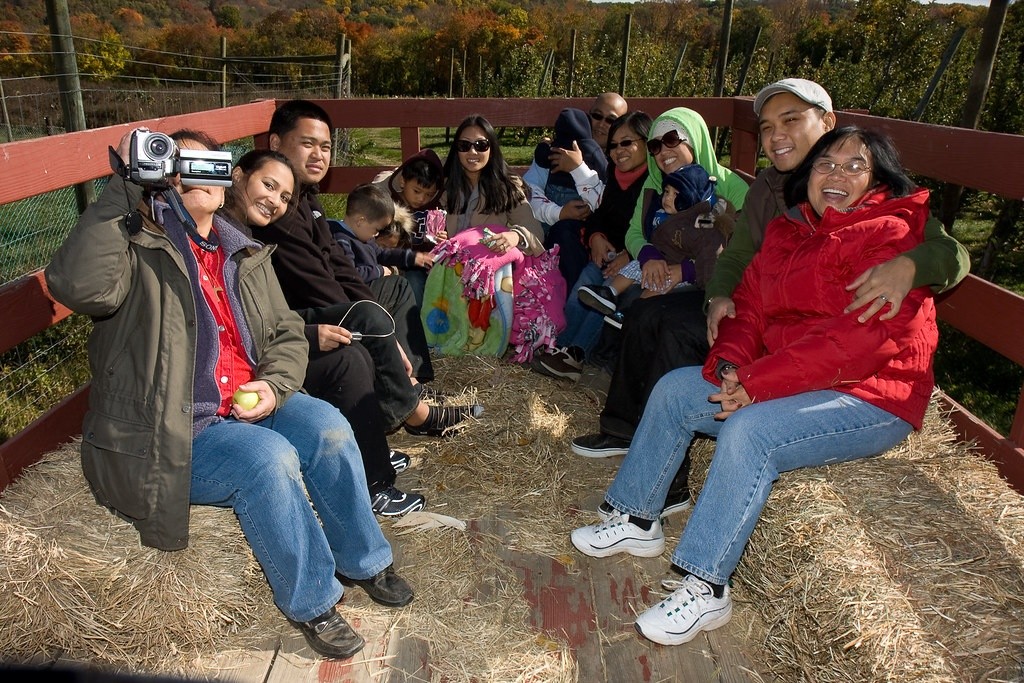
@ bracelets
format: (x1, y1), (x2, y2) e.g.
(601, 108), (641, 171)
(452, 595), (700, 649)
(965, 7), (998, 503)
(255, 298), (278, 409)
(726, 366), (732, 370)
(709, 297), (713, 303)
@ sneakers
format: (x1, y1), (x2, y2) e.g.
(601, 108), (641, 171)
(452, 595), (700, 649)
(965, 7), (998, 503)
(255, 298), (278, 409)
(603, 314), (624, 329)
(570, 510), (665, 558)
(571, 432), (630, 459)
(578, 285), (618, 314)
(389, 449), (410, 474)
(404, 405), (484, 437)
(530, 347), (584, 382)
(370, 486), (426, 516)
(598, 482), (692, 523)
(635, 576), (733, 646)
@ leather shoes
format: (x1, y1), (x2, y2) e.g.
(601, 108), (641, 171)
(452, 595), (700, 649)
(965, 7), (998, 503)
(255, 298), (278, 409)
(338, 565), (414, 606)
(287, 606), (365, 659)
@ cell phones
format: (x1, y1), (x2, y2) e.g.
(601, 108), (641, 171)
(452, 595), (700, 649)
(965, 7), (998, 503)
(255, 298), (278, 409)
(350, 332), (363, 340)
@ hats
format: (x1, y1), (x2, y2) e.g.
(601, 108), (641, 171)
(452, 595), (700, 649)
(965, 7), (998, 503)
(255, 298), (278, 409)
(653, 119), (695, 148)
(753, 78), (833, 117)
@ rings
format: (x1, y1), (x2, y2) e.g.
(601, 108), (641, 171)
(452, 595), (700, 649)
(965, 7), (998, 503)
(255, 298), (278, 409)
(503, 243), (506, 247)
(669, 275), (670, 278)
(881, 296), (886, 300)
(669, 279), (671, 282)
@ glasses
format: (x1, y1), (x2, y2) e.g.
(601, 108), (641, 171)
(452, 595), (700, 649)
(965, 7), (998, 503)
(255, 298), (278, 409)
(609, 138), (644, 149)
(648, 129), (685, 155)
(811, 158), (872, 176)
(454, 139), (490, 152)
(589, 112), (615, 125)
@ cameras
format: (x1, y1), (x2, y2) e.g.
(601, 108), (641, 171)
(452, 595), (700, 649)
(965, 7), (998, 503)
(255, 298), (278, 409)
(130, 128), (234, 191)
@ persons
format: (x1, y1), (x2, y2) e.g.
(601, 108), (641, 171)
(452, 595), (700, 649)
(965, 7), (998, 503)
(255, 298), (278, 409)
(326, 115), (544, 315)
(43, 127), (414, 659)
(572, 78), (970, 646)
(211, 100), (484, 517)
(529, 93), (750, 521)
